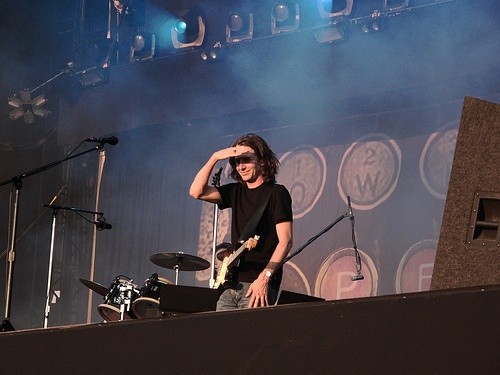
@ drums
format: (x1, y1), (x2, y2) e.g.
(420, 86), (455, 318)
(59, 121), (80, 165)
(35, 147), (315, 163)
(133, 277), (175, 318)
(97, 279), (141, 321)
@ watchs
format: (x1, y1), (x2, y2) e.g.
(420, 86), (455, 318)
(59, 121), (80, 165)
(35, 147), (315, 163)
(263, 269), (272, 278)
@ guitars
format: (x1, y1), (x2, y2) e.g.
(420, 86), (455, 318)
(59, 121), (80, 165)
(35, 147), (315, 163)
(214, 238), (258, 289)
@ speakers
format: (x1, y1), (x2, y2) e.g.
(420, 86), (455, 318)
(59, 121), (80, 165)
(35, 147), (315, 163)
(429, 96), (500, 291)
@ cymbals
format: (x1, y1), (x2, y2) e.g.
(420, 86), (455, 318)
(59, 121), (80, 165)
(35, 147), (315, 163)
(79, 278), (109, 296)
(150, 253), (211, 271)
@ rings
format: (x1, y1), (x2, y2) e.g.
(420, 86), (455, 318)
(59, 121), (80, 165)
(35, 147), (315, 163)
(233, 149), (237, 153)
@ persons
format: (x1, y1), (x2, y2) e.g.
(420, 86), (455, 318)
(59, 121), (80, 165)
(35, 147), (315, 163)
(189, 133), (293, 312)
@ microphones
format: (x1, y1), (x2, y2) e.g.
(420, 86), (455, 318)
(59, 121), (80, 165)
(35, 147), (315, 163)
(351, 275), (364, 281)
(94, 220), (112, 230)
(85, 136), (118, 146)
